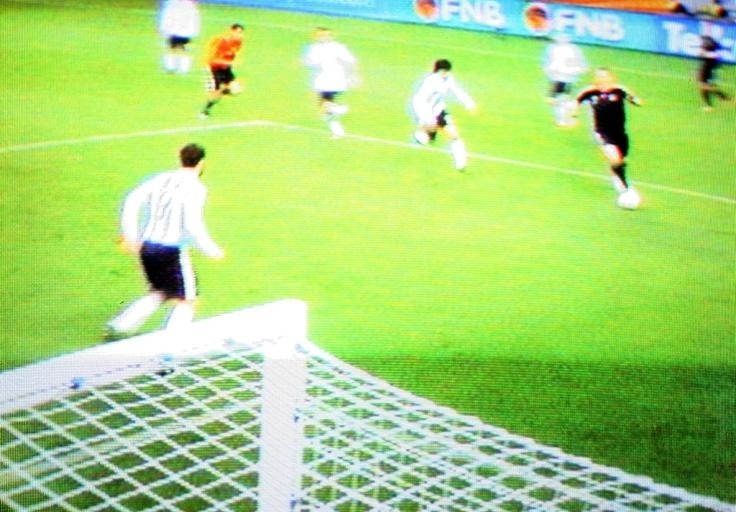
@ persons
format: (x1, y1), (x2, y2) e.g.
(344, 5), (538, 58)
(695, 30), (732, 112)
(296, 26), (362, 142)
(542, 30), (584, 128)
(196, 22), (246, 119)
(408, 57), (475, 176)
(571, 66), (646, 213)
(105, 143), (223, 380)
(155, 0), (201, 73)
